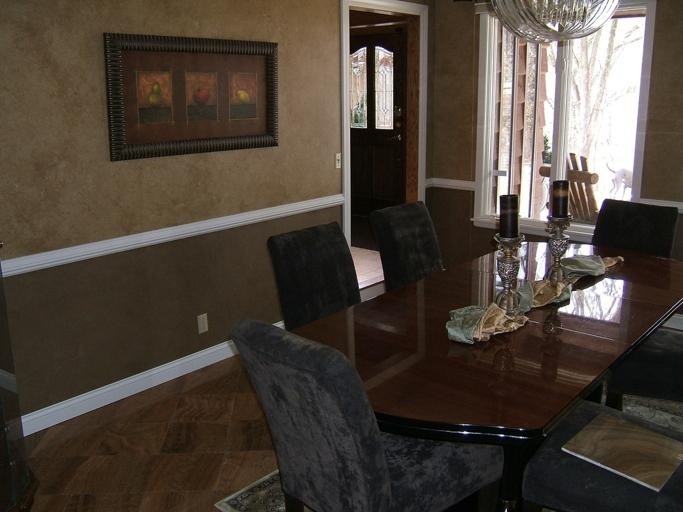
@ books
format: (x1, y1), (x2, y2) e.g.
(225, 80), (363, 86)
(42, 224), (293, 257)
(561, 412), (683, 492)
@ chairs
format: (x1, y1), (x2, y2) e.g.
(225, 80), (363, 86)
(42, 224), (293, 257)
(230, 318), (506, 512)
(592, 198), (680, 259)
(520, 399), (683, 512)
(604, 326), (683, 412)
(267, 220), (362, 330)
(368, 201), (444, 293)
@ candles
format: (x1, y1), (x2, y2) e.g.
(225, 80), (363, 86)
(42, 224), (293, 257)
(552, 180), (568, 218)
(500, 194), (518, 238)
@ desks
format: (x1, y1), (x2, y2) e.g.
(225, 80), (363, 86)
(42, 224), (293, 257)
(285, 241), (683, 512)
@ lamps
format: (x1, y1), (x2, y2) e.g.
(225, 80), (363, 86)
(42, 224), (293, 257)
(490, 0), (621, 43)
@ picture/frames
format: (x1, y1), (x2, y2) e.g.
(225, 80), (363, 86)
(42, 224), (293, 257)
(104, 32), (278, 162)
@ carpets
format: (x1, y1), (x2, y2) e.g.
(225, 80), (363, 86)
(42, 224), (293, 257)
(213, 466), (313, 512)
